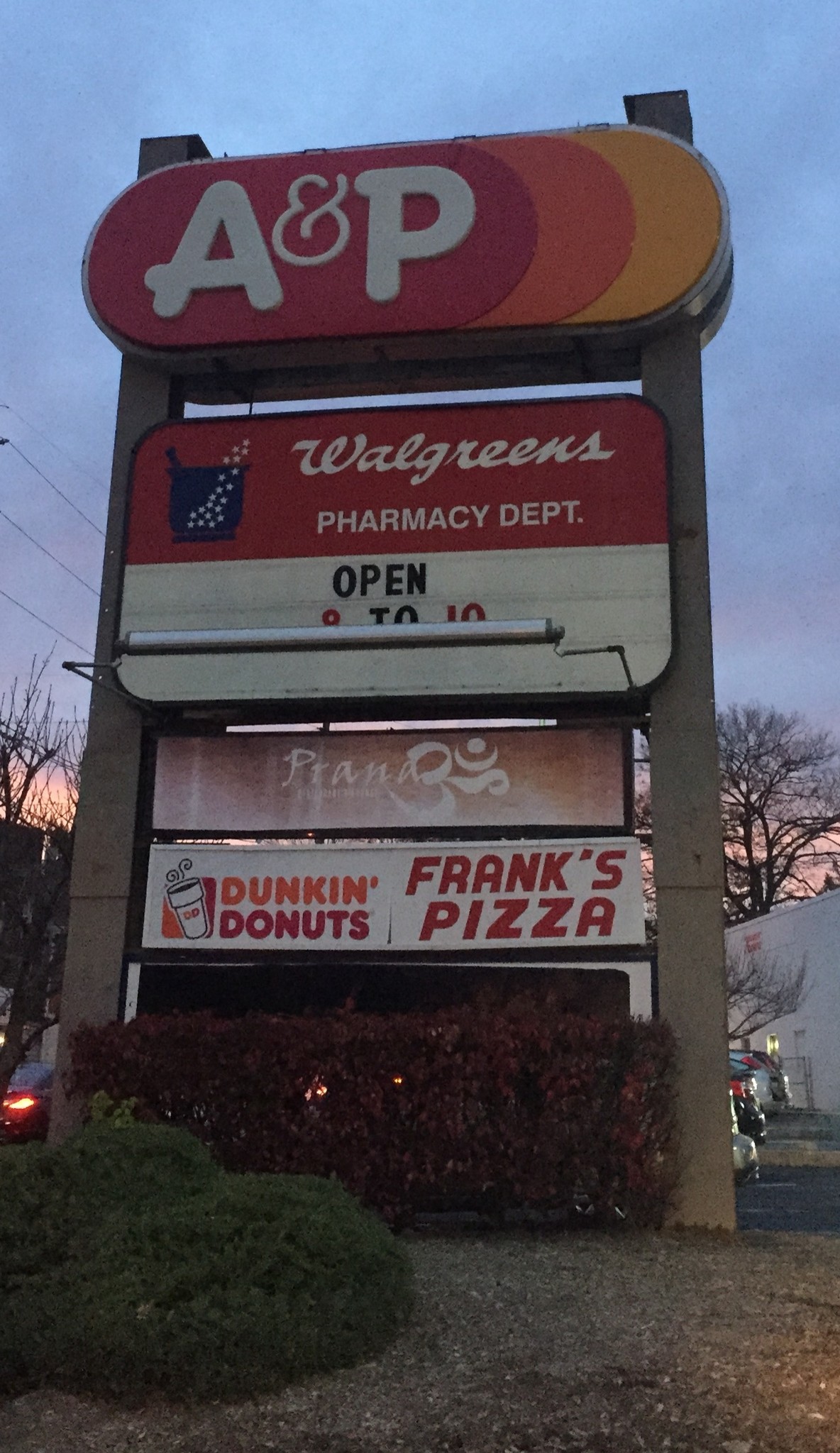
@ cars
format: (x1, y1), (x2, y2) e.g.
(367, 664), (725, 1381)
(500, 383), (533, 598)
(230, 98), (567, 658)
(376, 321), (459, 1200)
(729, 1058), (761, 1109)
(1, 1060), (54, 1143)
(729, 1088), (760, 1187)
(728, 1064), (767, 1146)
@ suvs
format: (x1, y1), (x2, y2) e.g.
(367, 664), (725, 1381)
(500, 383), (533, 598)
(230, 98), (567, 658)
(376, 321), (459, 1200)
(730, 1050), (794, 1118)
